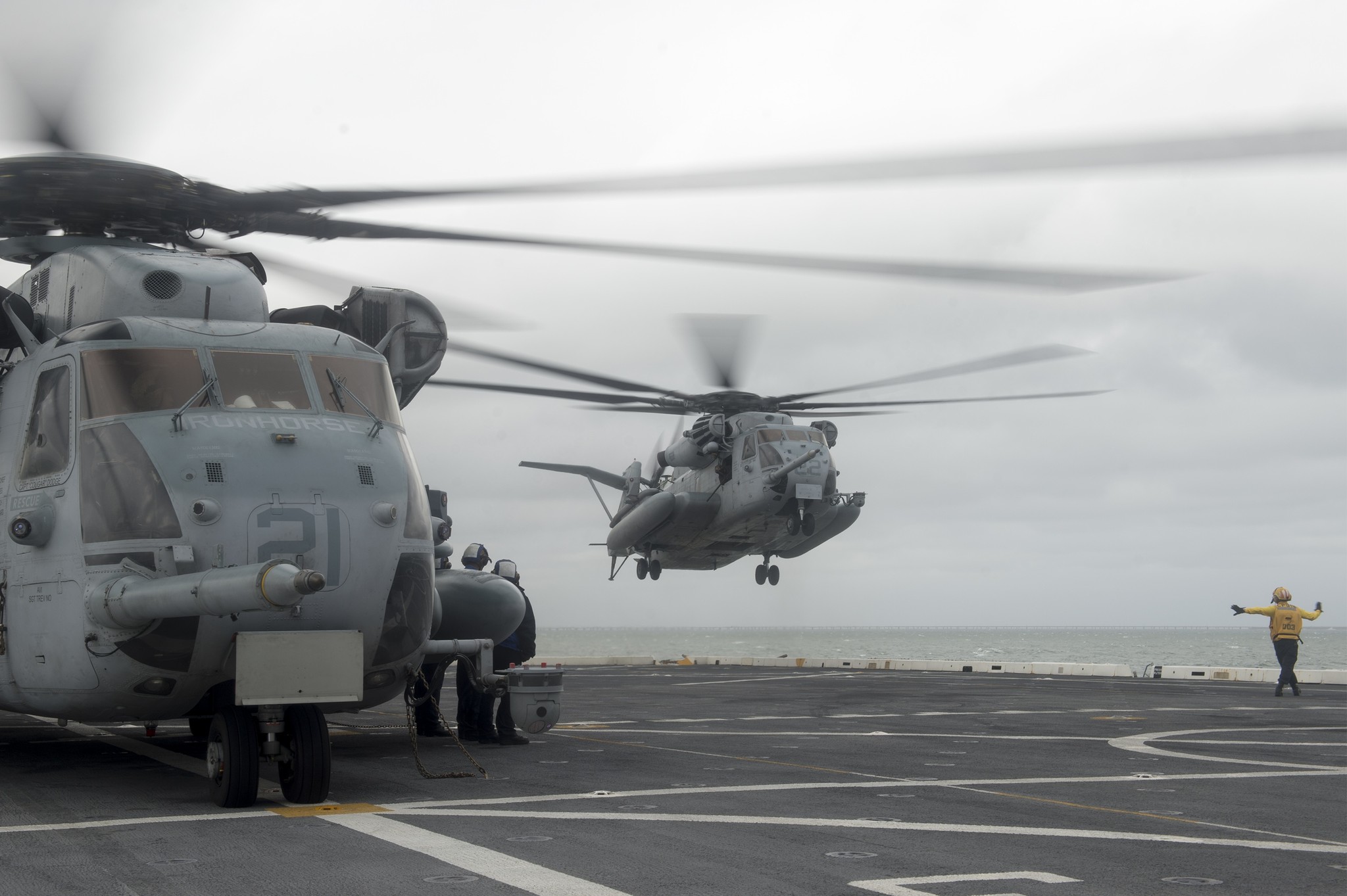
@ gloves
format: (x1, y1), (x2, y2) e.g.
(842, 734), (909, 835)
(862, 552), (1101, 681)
(1315, 602), (1323, 612)
(1231, 605), (1245, 616)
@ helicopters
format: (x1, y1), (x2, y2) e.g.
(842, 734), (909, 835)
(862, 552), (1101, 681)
(0, 0), (1342, 815)
(425, 307), (1107, 587)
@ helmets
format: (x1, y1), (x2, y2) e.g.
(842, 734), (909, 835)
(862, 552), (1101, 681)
(434, 557), (449, 569)
(1272, 587), (1291, 601)
(495, 560), (517, 581)
(461, 543), (489, 568)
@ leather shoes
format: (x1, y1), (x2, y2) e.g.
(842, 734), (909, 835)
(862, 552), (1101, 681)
(416, 722), (530, 745)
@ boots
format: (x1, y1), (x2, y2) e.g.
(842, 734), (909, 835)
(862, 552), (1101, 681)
(1274, 683), (1284, 696)
(1291, 683), (1302, 696)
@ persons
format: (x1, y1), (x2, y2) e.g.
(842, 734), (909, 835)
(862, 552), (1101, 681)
(412, 553), (452, 737)
(480, 560), (536, 744)
(1230, 586), (1323, 697)
(450, 542), (495, 741)
